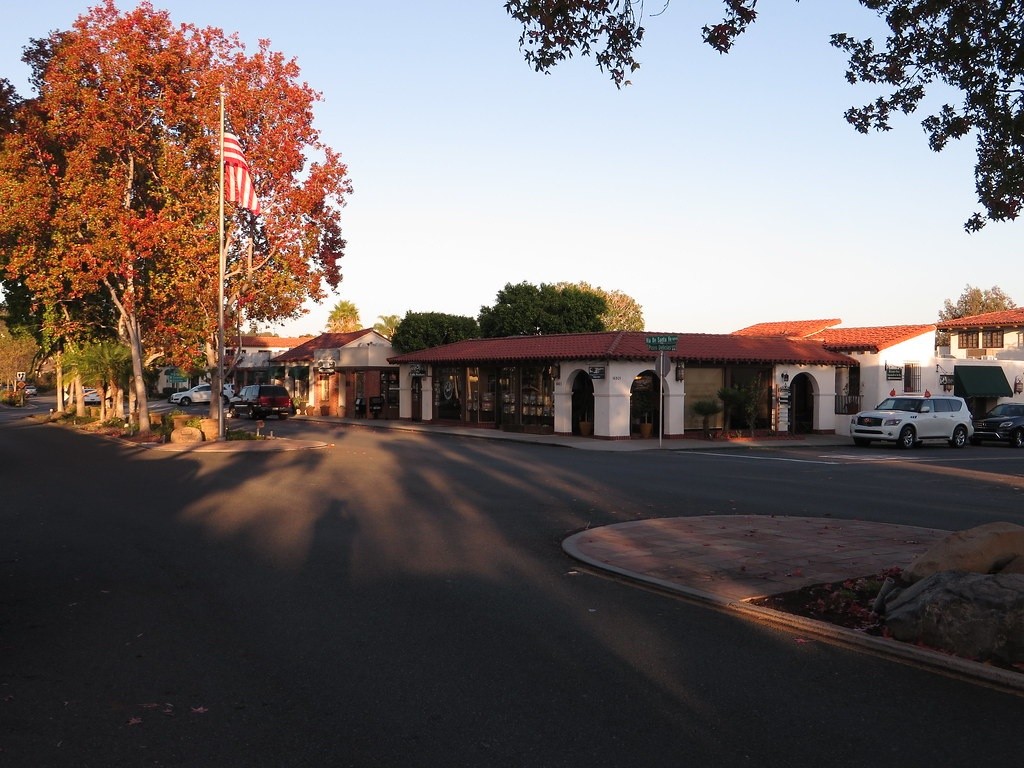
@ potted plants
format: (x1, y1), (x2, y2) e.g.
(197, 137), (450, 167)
(577, 375), (594, 437)
(634, 394), (662, 438)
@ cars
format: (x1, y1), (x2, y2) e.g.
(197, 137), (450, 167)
(969, 402), (1024, 448)
(24, 385), (38, 396)
(82, 388), (101, 405)
(228, 384), (293, 420)
(848, 393), (975, 449)
(170, 383), (235, 407)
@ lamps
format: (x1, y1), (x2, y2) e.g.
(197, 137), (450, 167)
(1014, 378), (1023, 395)
